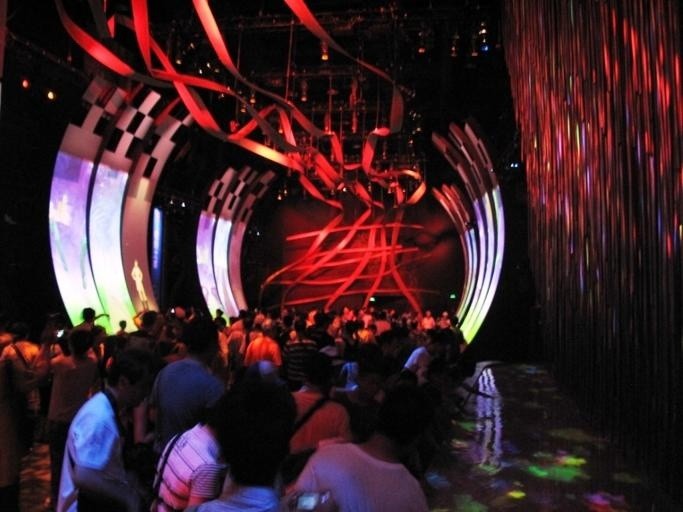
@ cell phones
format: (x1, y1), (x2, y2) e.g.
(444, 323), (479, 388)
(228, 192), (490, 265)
(55, 329), (65, 340)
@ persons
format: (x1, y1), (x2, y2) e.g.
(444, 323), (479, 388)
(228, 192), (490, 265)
(0, 303), (473, 512)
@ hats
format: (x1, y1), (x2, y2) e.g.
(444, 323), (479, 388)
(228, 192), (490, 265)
(246, 360), (287, 389)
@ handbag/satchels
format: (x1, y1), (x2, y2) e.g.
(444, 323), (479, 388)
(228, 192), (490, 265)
(269, 437), (315, 485)
(76, 490), (128, 512)
